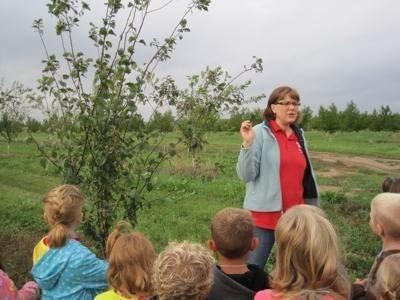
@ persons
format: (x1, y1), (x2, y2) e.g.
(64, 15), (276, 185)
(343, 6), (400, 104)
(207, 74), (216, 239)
(204, 205), (271, 300)
(381, 175), (400, 193)
(93, 218), (157, 300)
(236, 85), (320, 269)
(254, 203), (352, 300)
(30, 183), (110, 300)
(0, 262), (42, 300)
(351, 191), (400, 300)
(146, 237), (215, 300)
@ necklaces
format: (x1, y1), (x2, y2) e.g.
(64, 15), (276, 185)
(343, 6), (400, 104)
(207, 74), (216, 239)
(219, 264), (248, 268)
